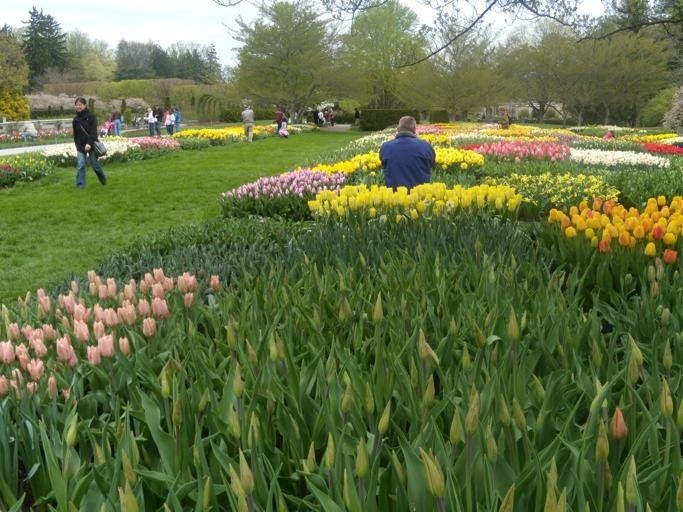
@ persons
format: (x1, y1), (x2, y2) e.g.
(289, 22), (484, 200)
(240, 105), (255, 143)
(377, 115), (434, 192)
(70, 96), (107, 187)
(271, 103), (360, 136)
(21, 121), (38, 142)
(101, 103), (181, 137)
(497, 104), (510, 128)
(602, 129), (614, 140)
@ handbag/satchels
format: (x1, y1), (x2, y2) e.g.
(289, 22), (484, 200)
(92, 141), (106, 156)
